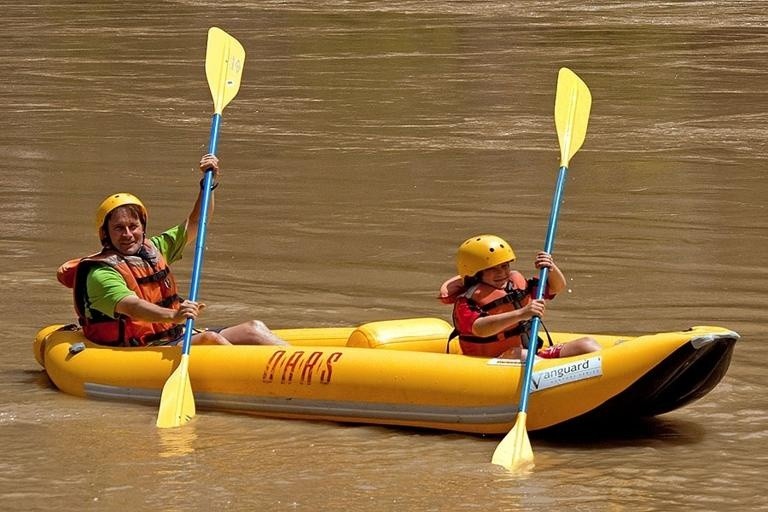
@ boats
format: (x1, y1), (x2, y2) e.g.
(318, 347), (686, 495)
(33, 316), (739, 439)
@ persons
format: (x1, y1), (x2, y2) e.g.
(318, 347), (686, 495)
(56, 152), (295, 347)
(437, 234), (601, 364)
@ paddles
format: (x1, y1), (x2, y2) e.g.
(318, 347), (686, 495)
(158, 25), (246, 435)
(492, 68), (592, 465)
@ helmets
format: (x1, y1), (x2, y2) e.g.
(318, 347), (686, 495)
(94, 193), (148, 247)
(455, 235), (517, 288)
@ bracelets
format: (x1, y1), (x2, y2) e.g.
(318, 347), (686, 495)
(200, 178), (219, 191)
